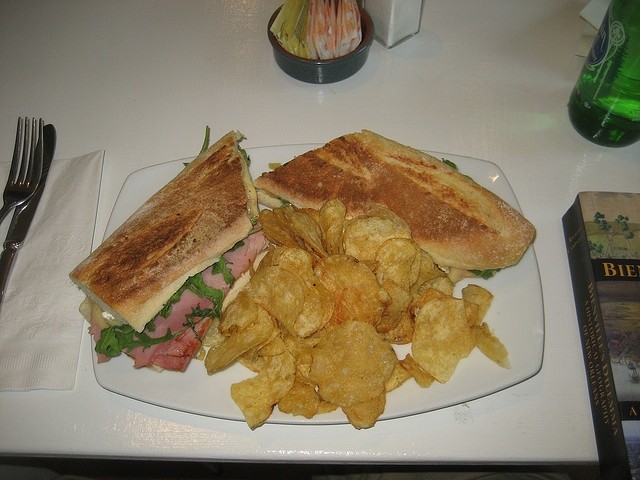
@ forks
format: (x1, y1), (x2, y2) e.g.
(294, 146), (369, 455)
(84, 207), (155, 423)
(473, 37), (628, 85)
(0, 116), (45, 224)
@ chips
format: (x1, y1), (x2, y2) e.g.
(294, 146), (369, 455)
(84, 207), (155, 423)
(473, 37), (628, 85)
(204, 198), (511, 430)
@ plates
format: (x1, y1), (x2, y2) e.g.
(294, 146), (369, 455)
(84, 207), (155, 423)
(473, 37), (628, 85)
(89, 142), (546, 425)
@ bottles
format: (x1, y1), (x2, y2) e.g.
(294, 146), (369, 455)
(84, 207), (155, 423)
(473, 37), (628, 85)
(363, 0), (424, 50)
(568, 0), (640, 148)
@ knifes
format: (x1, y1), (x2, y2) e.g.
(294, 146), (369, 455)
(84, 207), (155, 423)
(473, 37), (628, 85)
(0, 123), (57, 306)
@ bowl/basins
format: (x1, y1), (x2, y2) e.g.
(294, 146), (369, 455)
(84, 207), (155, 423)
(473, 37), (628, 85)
(267, 3), (374, 84)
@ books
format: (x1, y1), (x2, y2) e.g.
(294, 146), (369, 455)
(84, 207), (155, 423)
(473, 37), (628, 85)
(562, 191), (640, 480)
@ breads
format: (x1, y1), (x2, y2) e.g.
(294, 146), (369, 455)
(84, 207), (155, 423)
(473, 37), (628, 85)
(251, 128), (536, 284)
(69, 126), (272, 373)
